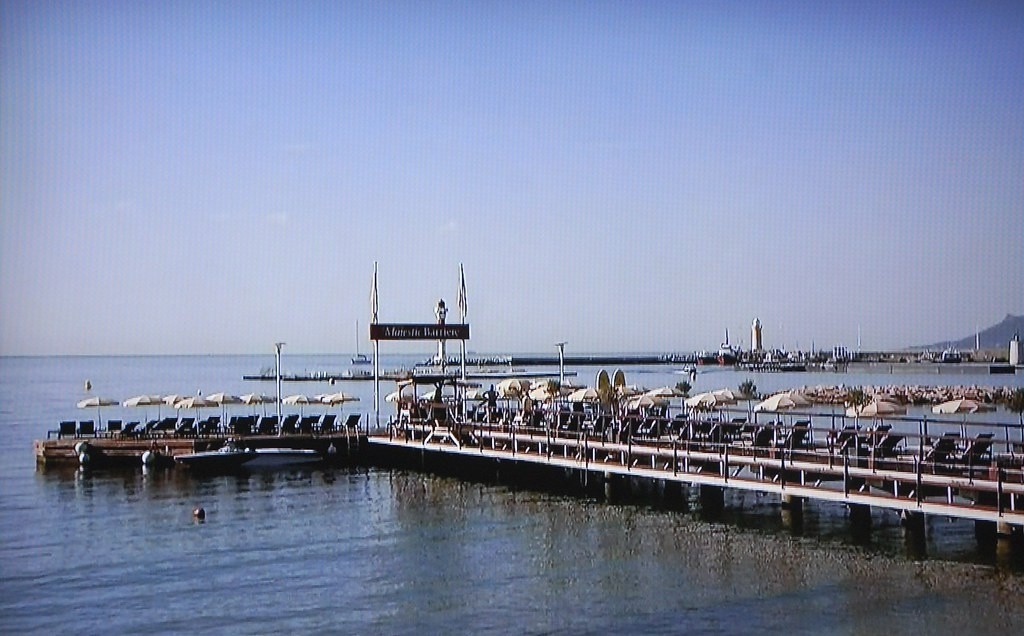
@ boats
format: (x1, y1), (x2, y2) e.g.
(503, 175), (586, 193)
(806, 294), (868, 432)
(174, 441), (325, 473)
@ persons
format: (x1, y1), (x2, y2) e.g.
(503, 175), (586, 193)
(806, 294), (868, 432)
(482, 383), (499, 423)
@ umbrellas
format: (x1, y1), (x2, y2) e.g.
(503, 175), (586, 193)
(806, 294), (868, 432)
(684, 387), (756, 441)
(754, 393), (814, 424)
(845, 397), (907, 426)
(461, 379), (575, 424)
(122, 388), (276, 437)
(931, 396), (997, 447)
(77, 397), (119, 437)
(555, 384), (692, 421)
(419, 392), (454, 402)
(282, 390), (360, 420)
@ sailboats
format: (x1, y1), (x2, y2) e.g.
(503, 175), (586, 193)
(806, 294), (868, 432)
(352, 320), (371, 364)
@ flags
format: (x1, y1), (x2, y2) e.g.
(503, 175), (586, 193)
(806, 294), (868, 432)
(457, 265), (467, 317)
(370, 270), (379, 323)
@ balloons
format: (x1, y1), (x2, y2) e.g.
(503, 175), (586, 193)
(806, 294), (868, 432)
(384, 390), (413, 408)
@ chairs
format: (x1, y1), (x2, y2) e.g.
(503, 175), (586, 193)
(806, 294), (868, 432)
(57, 414), (360, 442)
(391, 398), (995, 494)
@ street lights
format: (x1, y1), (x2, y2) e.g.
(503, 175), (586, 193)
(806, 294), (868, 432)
(556, 342), (567, 408)
(274, 342), (286, 437)
